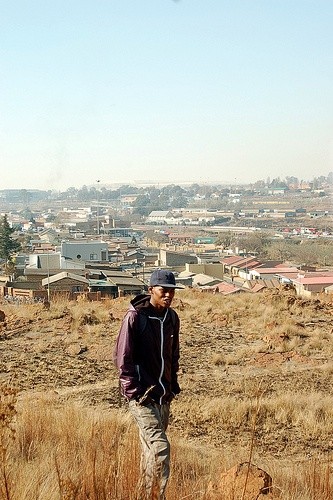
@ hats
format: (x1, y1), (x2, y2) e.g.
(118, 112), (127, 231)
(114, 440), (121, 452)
(150, 270), (185, 289)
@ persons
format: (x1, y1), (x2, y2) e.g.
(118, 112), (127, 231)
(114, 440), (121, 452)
(111, 269), (182, 500)
